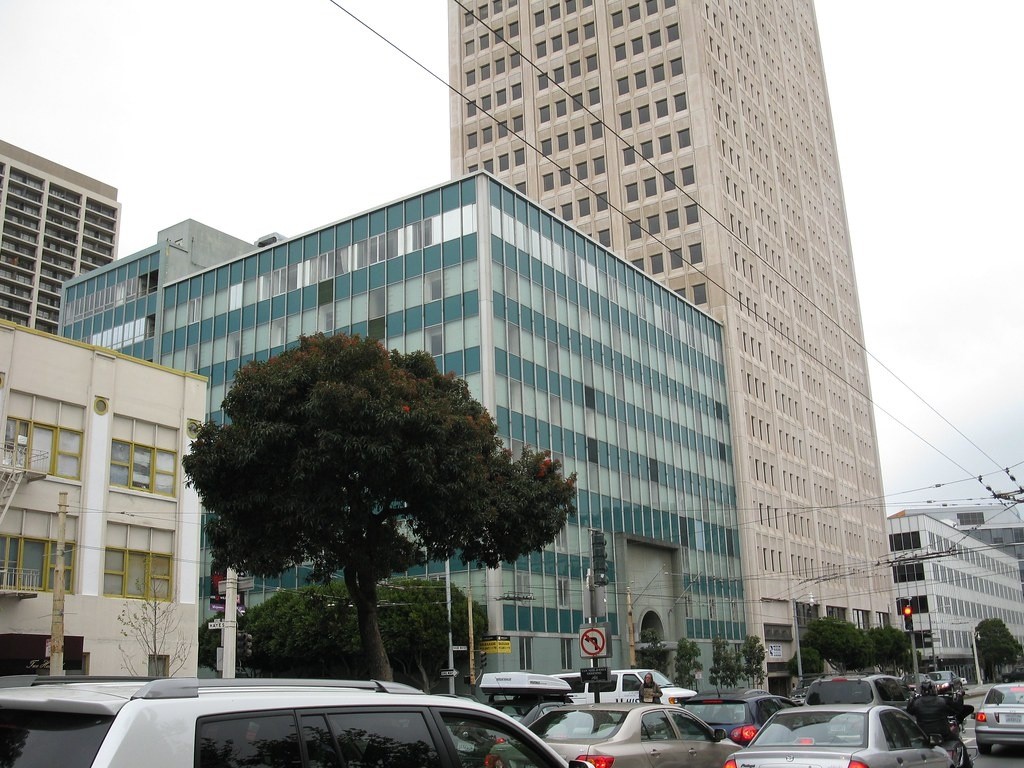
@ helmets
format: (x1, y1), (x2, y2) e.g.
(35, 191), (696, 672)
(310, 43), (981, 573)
(921, 680), (936, 697)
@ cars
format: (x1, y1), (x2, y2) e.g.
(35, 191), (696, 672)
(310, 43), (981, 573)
(472, 668), (1024, 768)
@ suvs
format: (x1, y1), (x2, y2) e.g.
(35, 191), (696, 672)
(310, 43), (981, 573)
(1, 672), (595, 768)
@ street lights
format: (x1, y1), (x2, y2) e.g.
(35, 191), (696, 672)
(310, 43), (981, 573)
(973, 631), (983, 684)
(928, 605), (951, 671)
(792, 592), (815, 680)
(887, 596), (912, 625)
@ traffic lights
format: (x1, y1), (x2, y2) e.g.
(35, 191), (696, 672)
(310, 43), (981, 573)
(903, 606), (913, 630)
(593, 533), (609, 586)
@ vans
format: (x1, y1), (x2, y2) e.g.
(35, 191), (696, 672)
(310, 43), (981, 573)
(552, 670), (698, 710)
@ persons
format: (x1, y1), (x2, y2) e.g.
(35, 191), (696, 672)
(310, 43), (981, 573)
(639, 672), (662, 703)
(906, 679), (974, 768)
